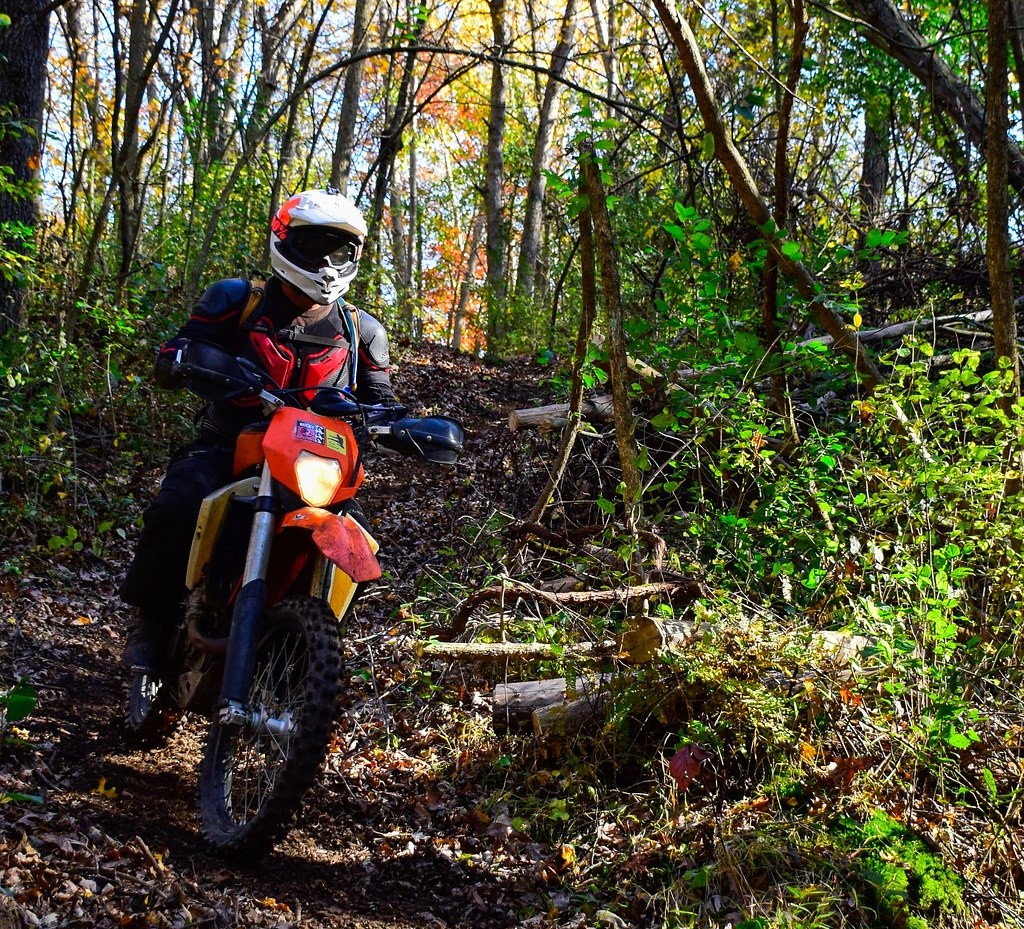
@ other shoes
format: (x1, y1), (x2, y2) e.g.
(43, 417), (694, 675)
(125, 606), (163, 673)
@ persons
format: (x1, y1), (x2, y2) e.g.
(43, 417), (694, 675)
(112, 188), (404, 665)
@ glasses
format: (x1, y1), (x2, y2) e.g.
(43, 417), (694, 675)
(270, 213), (363, 271)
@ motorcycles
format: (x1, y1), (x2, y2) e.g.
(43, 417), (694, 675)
(124, 327), (466, 851)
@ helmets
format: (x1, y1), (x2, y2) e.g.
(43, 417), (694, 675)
(270, 186), (368, 305)
